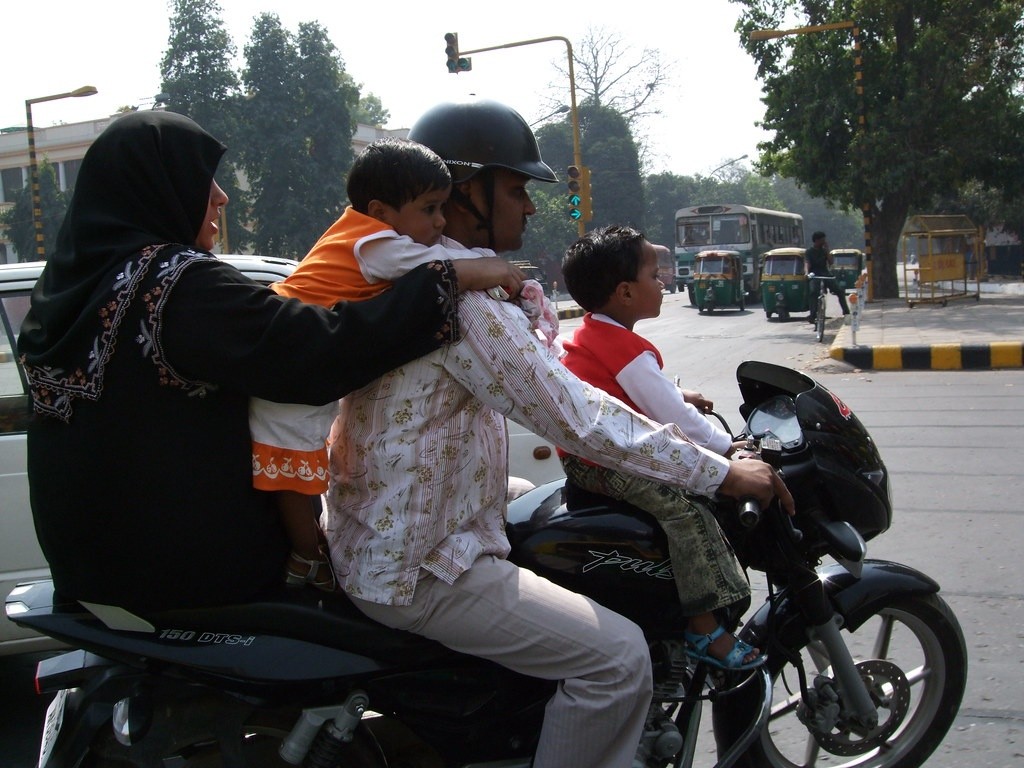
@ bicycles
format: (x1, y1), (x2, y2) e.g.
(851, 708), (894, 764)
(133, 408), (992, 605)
(805, 270), (837, 342)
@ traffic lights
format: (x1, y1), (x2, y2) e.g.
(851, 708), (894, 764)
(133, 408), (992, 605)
(581, 166), (594, 223)
(458, 56), (472, 71)
(567, 165), (585, 223)
(445, 31), (460, 73)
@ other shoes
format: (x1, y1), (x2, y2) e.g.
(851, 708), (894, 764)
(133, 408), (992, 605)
(843, 310), (849, 315)
(809, 315), (816, 322)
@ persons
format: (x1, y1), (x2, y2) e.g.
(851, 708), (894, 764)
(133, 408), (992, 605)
(805, 231), (851, 324)
(16, 99), (797, 768)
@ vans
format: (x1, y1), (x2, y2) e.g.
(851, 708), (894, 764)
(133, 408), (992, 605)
(0, 252), (303, 659)
(650, 244), (677, 294)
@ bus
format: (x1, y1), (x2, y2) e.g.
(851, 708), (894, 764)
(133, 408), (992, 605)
(674, 203), (806, 293)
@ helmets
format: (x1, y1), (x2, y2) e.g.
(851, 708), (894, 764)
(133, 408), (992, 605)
(406, 92), (559, 184)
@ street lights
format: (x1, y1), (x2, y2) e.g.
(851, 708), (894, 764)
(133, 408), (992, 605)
(25, 86), (99, 259)
(749, 19), (875, 303)
(705, 154), (748, 203)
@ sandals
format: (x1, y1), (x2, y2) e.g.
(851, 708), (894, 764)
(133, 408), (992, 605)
(283, 542), (341, 593)
(684, 625), (768, 671)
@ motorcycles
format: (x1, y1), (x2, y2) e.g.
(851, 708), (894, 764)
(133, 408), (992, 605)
(0, 359), (968, 767)
(692, 249), (749, 315)
(757, 247), (815, 323)
(829, 247), (865, 296)
